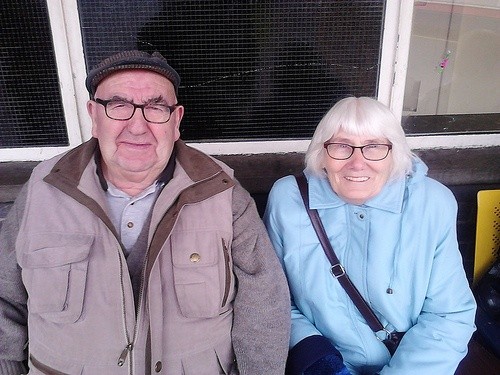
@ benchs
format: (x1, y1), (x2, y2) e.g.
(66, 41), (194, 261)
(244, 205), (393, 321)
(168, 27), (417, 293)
(0, 144), (500, 375)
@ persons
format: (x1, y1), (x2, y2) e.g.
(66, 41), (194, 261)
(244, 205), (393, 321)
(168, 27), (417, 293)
(263, 97), (477, 375)
(0, 50), (292, 375)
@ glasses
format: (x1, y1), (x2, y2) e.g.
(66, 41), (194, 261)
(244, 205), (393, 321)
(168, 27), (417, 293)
(323, 142), (393, 162)
(91, 97), (179, 124)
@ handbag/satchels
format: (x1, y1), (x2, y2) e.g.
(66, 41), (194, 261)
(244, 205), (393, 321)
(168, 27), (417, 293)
(382, 326), (500, 375)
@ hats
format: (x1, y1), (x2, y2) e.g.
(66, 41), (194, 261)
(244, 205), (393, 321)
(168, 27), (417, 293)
(85, 51), (180, 92)
